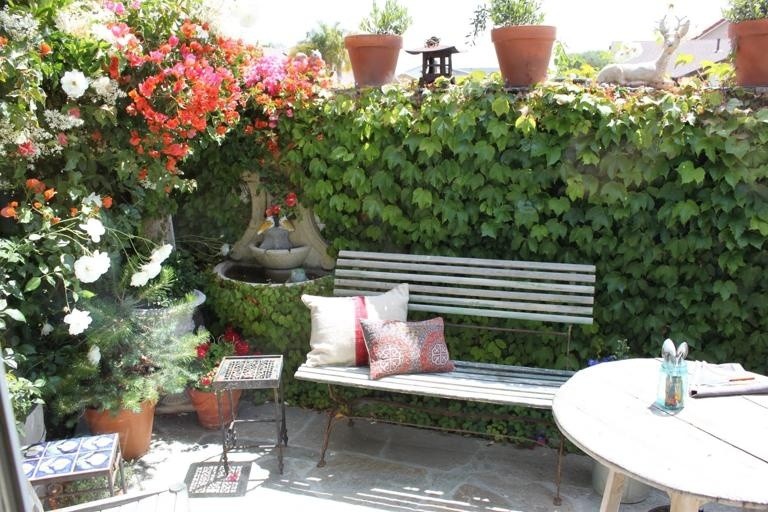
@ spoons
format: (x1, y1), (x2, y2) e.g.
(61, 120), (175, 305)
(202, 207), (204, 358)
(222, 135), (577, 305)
(661, 338), (676, 361)
(676, 340), (689, 363)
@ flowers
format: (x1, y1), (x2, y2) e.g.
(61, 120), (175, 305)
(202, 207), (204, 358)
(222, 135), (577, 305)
(187, 325), (249, 395)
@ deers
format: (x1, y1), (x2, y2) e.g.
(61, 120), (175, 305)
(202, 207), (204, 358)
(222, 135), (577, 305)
(594, 16), (691, 88)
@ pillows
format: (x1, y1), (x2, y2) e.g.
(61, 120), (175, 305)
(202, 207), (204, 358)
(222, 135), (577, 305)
(296, 278), (410, 370)
(356, 315), (457, 381)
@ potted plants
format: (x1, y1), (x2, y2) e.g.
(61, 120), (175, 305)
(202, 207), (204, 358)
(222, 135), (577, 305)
(342, 0), (413, 88)
(39, 291), (209, 465)
(462, 0), (558, 86)
(4, 366), (65, 452)
(717, 0), (767, 86)
(114, 242), (206, 351)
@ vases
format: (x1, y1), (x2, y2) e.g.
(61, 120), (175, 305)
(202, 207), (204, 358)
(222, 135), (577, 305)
(188, 384), (243, 430)
(206, 259), (337, 403)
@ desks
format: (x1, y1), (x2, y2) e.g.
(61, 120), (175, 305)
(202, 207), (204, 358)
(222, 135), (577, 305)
(208, 353), (291, 483)
(549, 353), (768, 512)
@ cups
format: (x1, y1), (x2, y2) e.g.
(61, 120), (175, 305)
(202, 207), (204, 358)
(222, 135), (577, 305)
(660, 360), (689, 409)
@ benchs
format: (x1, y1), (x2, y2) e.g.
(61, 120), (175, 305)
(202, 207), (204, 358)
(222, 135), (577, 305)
(288, 248), (600, 508)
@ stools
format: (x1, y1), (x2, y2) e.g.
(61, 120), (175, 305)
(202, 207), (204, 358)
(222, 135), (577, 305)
(15, 431), (128, 505)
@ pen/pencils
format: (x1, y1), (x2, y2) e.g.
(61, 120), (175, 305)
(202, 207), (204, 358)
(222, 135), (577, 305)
(730, 378), (754, 382)
